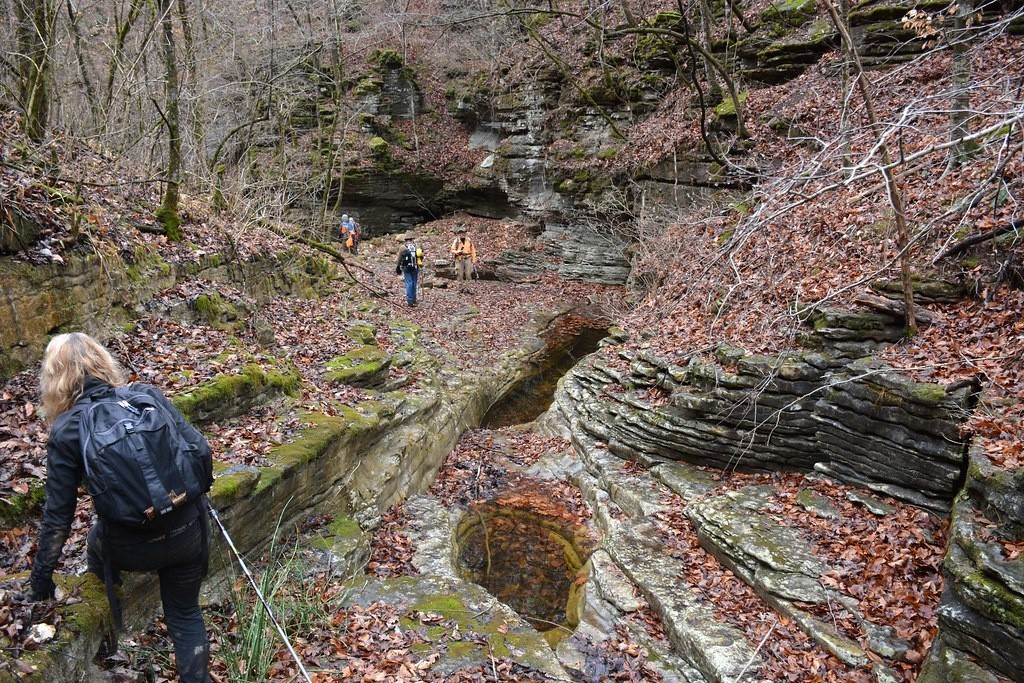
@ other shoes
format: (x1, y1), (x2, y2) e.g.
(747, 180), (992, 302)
(467, 288), (475, 295)
(456, 289), (464, 293)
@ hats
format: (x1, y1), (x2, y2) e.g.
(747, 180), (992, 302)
(457, 226), (467, 233)
(404, 233), (415, 239)
(342, 214), (348, 221)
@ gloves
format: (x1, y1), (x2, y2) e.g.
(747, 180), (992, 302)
(396, 266), (402, 275)
(29, 577), (56, 602)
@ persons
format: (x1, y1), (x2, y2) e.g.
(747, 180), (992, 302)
(29, 333), (214, 683)
(396, 236), (424, 307)
(339, 214), (360, 256)
(451, 229), (477, 294)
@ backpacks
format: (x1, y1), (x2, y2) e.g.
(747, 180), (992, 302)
(71, 381), (209, 545)
